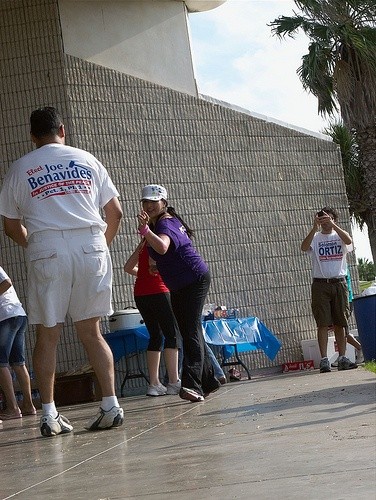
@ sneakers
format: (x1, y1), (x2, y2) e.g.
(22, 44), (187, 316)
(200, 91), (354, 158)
(180, 386), (204, 402)
(146, 383), (167, 396)
(166, 379), (181, 394)
(338, 357), (357, 370)
(40, 413), (73, 436)
(83, 406), (123, 430)
(319, 359), (331, 372)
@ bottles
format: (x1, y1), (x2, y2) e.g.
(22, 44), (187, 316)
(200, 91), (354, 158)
(11, 362), (40, 401)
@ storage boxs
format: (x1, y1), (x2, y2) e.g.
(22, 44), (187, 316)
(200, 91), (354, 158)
(301, 333), (356, 370)
(55, 372), (102, 409)
(282, 360), (313, 373)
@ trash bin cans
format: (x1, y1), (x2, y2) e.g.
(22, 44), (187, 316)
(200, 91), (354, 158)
(352, 295), (375, 363)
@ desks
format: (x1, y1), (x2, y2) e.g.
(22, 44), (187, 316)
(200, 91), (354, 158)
(101, 316), (282, 398)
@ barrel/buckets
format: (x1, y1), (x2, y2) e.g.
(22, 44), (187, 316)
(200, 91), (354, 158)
(352, 292), (376, 362)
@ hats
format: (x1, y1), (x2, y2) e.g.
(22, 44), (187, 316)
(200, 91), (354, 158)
(139, 185), (167, 201)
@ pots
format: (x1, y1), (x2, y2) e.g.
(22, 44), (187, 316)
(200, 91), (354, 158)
(108, 306), (146, 332)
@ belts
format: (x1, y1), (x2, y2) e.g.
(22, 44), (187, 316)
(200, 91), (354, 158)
(314, 277), (345, 282)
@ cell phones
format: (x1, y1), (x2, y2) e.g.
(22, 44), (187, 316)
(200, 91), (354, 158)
(318, 211), (323, 220)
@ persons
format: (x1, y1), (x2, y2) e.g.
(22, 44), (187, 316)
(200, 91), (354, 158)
(136, 184), (221, 402)
(0, 263), (37, 420)
(0, 107), (124, 438)
(330, 268), (365, 368)
(302, 206), (356, 373)
(204, 338), (227, 384)
(124, 237), (183, 397)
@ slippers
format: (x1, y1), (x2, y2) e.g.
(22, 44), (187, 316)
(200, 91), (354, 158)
(0, 412), (22, 420)
(23, 410), (36, 416)
